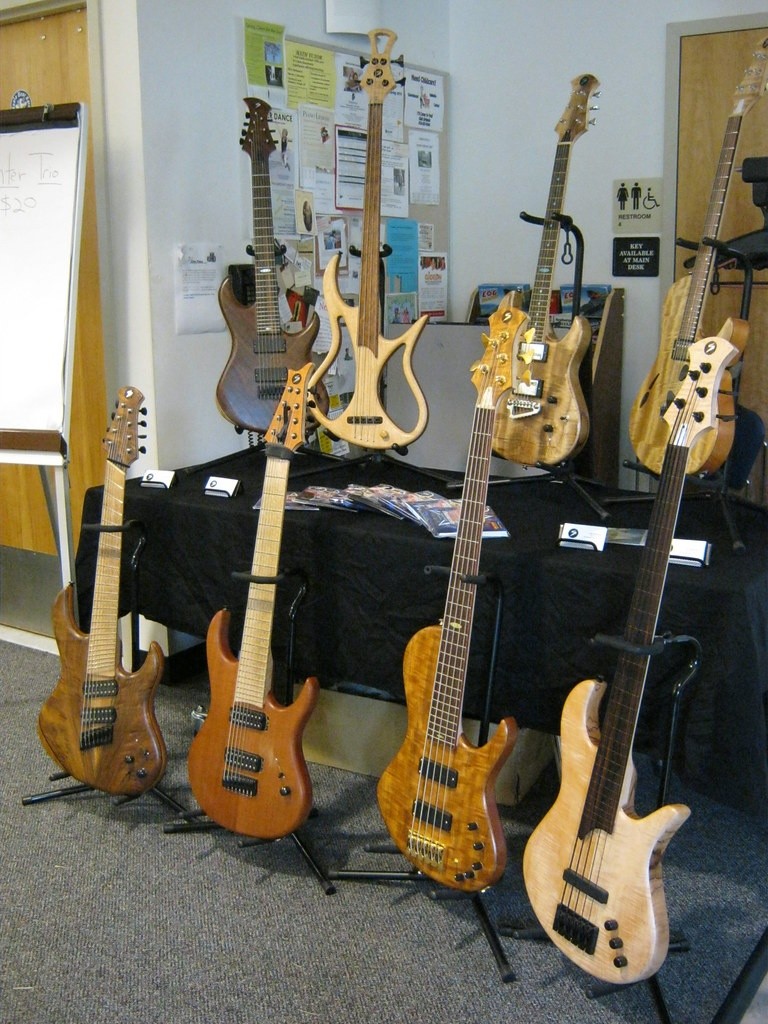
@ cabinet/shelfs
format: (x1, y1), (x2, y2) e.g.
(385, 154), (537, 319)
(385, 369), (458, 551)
(465, 283), (625, 487)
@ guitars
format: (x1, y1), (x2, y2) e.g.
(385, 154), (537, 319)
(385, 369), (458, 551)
(373, 290), (531, 894)
(628, 34), (768, 482)
(36, 385), (169, 798)
(214, 96), (332, 438)
(522, 336), (741, 987)
(304, 28), (432, 456)
(491, 73), (604, 470)
(186, 360), (323, 841)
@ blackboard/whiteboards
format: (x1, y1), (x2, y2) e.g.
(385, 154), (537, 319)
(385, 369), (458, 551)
(0, 100), (87, 467)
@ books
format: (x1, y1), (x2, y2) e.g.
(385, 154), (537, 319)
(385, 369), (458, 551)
(548, 311), (580, 341)
(584, 315), (603, 353)
(253, 483), (510, 539)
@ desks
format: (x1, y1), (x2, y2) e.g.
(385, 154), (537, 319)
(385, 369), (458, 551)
(76, 440), (767, 809)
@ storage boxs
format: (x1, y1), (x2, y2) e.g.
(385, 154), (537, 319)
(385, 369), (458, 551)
(291, 684), (555, 807)
